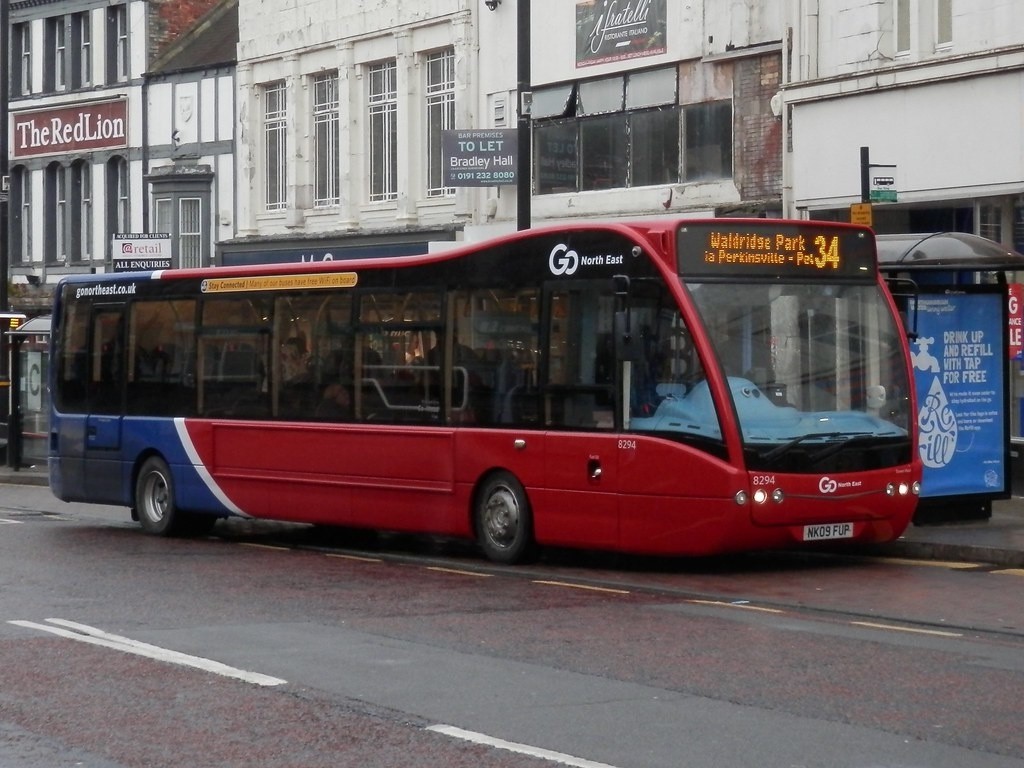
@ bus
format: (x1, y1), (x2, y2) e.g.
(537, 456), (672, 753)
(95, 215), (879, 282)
(49, 215), (921, 567)
